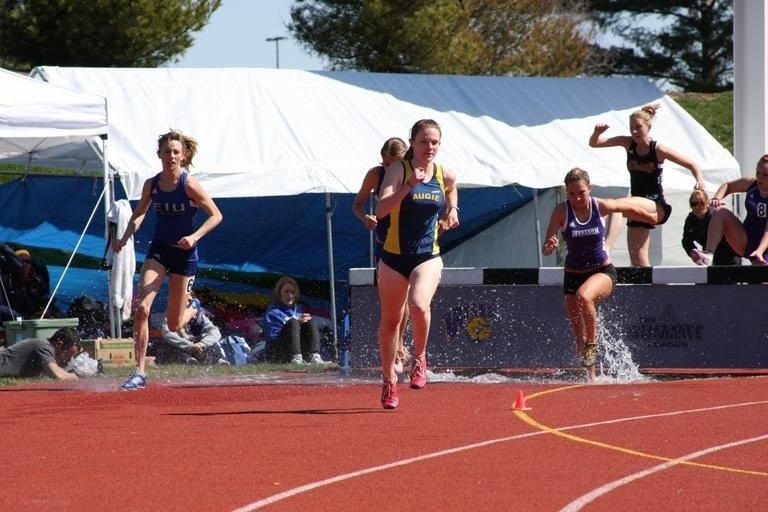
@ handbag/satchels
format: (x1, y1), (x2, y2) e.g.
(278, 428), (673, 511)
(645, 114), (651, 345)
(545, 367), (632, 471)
(220, 335), (252, 366)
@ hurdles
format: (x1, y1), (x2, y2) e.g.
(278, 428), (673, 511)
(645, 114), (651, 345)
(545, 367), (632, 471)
(348, 267), (767, 370)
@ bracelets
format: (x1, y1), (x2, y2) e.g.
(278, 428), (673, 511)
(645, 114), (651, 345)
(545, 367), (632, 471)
(447, 204), (462, 213)
(404, 178), (414, 190)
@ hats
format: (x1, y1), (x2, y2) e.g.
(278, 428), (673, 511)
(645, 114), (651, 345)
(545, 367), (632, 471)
(14, 249), (30, 255)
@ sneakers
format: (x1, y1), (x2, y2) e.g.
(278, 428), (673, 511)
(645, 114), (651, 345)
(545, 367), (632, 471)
(582, 343), (600, 367)
(123, 373), (146, 389)
(691, 247), (713, 266)
(311, 354), (333, 365)
(291, 356), (309, 365)
(189, 299), (203, 336)
(381, 379), (399, 408)
(410, 356), (428, 388)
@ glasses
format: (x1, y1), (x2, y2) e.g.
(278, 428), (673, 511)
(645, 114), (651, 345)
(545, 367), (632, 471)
(690, 203), (700, 207)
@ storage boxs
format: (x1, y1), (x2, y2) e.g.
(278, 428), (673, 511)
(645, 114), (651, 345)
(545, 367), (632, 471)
(81, 336), (138, 370)
(2, 316), (80, 349)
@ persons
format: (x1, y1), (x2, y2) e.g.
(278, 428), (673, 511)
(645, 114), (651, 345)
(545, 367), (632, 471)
(680, 182), (745, 266)
(111, 132), (225, 392)
(686, 154), (767, 269)
(351, 136), (411, 376)
(585, 105), (707, 270)
(2, 243), (324, 381)
(375, 118), (461, 411)
(543, 167), (660, 384)
